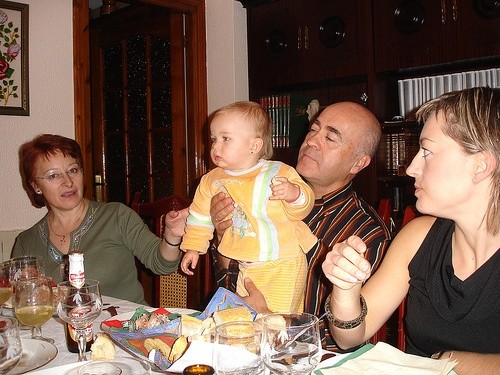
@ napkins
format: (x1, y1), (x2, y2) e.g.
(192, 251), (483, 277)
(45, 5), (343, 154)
(315, 341), (457, 375)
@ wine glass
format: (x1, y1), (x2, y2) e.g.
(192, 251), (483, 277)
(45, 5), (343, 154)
(56, 278), (103, 362)
(12, 276), (56, 344)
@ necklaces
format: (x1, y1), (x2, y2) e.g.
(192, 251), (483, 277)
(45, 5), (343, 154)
(49, 199), (85, 244)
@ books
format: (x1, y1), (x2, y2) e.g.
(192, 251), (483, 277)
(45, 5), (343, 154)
(258, 68), (500, 221)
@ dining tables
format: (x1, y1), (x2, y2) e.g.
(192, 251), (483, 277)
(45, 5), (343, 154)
(19, 288), (337, 375)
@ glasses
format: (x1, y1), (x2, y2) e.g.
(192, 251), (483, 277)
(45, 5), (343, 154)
(33, 167), (84, 184)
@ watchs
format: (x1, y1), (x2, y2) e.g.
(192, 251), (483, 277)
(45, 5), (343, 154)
(430, 350), (446, 358)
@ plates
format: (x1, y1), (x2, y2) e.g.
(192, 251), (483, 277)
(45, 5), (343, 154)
(101, 308), (201, 330)
(4, 338), (58, 375)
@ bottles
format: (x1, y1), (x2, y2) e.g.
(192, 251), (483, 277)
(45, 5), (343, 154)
(64, 251), (93, 354)
(183, 364), (215, 375)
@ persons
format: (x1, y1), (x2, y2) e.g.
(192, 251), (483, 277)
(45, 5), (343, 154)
(322, 87), (500, 375)
(179, 101), (316, 330)
(11, 134), (188, 309)
(209, 101), (392, 355)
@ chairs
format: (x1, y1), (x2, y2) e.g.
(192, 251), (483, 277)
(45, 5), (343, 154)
(137, 194), (213, 313)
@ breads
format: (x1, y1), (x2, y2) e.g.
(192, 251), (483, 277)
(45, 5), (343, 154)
(89, 332), (115, 359)
(144, 335), (188, 363)
(179, 306), (286, 352)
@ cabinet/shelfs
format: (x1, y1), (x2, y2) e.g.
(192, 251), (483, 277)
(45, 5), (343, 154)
(240, 0), (500, 221)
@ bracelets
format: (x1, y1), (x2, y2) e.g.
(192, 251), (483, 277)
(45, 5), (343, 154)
(163, 235), (181, 247)
(324, 293), (367, 329)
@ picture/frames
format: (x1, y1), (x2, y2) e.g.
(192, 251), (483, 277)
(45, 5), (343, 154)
(0, 0), (30, 116)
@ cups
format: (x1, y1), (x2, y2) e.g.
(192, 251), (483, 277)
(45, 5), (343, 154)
(0, 262), (13, 316)
(212, 320), (269, 375)
(0, 316), (22, 374)
(262, 312), (322, 375)
(78, 356), (152, 375)
(7, 256), (45, 288)
